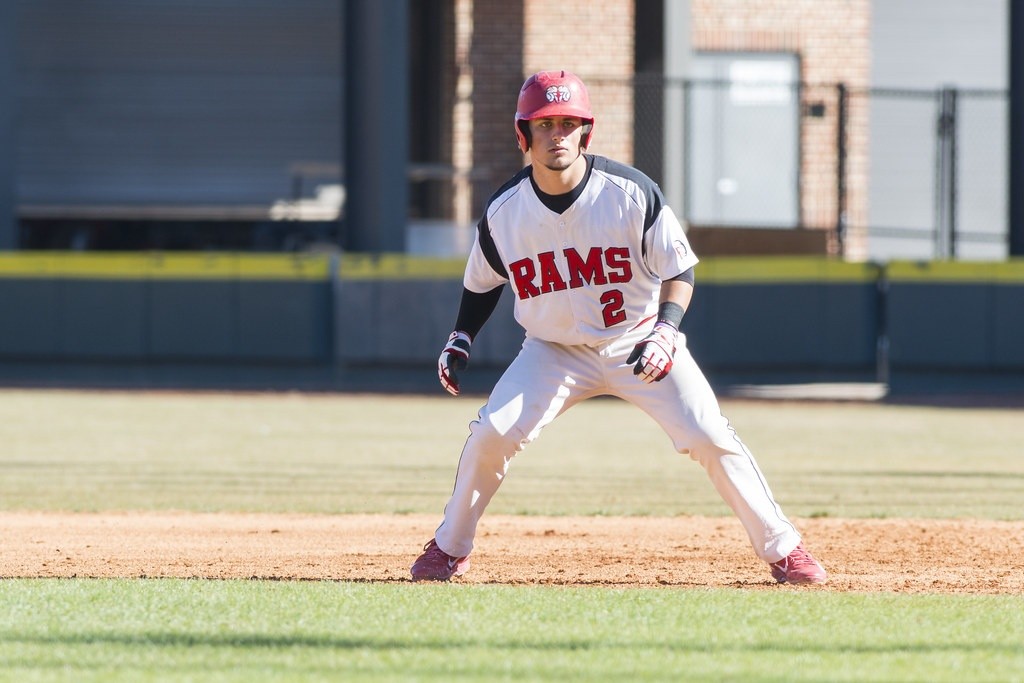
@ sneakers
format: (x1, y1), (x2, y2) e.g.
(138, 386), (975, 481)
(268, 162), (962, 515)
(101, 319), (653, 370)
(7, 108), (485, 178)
(769, 538), (828, 587)
(409, 537), (470, 580)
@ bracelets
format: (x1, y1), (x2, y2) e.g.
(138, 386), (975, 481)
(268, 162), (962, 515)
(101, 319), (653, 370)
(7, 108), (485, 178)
(658, 302), (684, 327)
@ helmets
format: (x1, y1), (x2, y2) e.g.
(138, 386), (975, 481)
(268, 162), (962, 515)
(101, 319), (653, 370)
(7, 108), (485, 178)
(514, 70), (594, 154)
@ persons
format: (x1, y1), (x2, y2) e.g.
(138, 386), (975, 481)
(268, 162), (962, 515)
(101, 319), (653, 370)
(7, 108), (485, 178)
(411, 72), (827, 586)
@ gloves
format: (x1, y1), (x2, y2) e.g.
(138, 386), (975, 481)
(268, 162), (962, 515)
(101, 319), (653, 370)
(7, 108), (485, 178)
(438, 330), (472, 396)
(627, 323), (678, 383)
